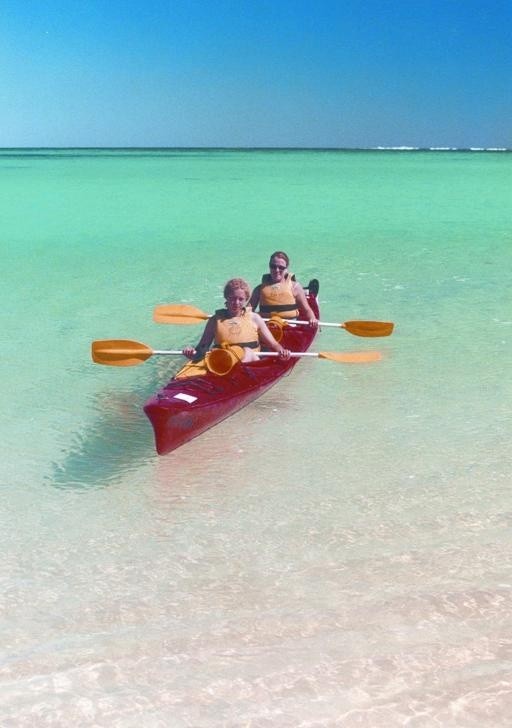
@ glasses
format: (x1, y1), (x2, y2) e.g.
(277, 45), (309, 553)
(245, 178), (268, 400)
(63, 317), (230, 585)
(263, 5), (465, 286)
(270, 263), (287, 270)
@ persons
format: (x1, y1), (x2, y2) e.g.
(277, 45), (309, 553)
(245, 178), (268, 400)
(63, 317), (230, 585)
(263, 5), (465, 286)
(182, 278), (292, 364)
(246, 251), (319, 329)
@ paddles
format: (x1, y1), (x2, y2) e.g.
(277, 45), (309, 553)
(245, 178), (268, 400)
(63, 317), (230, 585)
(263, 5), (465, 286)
(154, 303), (394, 337)
(92, 339), (383, 367)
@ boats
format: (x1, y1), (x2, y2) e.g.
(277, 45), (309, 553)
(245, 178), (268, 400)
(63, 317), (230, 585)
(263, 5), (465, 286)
(143, 276), (321, 455)
